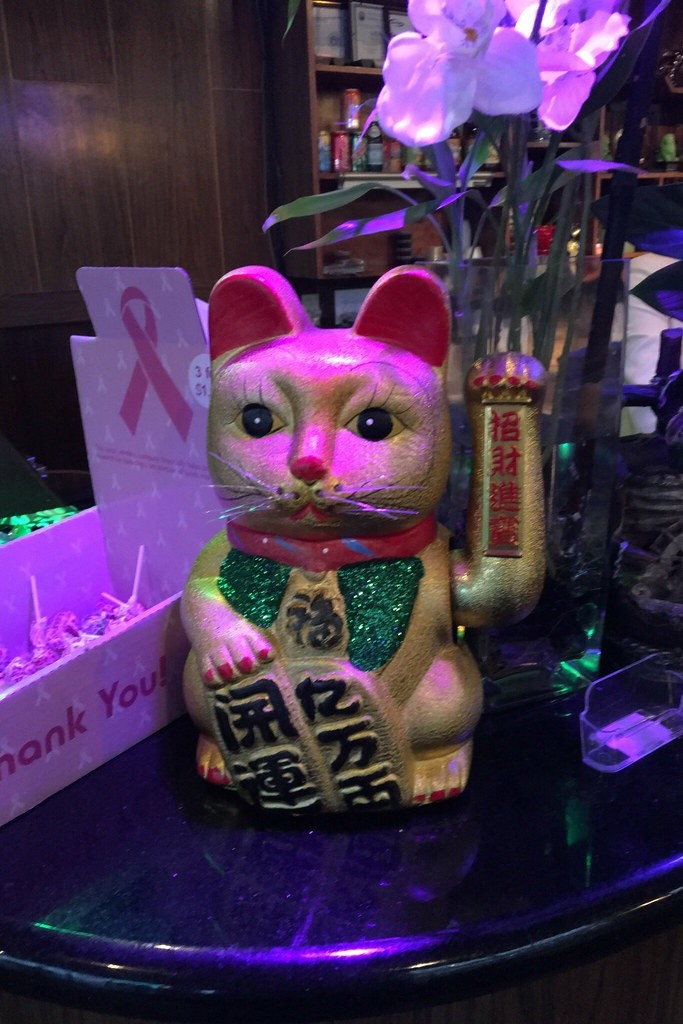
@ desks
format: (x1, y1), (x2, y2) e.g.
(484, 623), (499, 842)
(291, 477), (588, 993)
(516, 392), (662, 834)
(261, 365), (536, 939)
(1, 588), (682, 1024)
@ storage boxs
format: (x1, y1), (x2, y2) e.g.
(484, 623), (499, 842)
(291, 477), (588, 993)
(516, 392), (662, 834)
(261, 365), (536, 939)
(1, 267), (225, 829)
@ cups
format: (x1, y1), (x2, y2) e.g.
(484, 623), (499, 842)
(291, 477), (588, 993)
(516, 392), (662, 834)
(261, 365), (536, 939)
(427, 245), (443, 263)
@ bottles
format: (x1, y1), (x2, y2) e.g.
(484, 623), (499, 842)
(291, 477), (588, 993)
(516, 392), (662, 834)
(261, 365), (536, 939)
(368, 120), (384, 172)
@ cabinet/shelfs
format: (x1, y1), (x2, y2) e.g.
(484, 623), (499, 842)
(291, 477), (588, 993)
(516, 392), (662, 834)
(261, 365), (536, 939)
(268, 0), (682, 330)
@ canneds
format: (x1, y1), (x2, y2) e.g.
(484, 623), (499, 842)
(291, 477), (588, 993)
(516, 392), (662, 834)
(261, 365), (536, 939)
(342, 88), (361, 130)
(318, 121), (403, 175)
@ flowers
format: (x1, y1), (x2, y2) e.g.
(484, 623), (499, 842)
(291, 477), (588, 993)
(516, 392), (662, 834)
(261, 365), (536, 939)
(259, 0), (683, 648)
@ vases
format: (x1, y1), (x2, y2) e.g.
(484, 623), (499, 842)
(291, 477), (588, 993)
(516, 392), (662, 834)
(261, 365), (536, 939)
(437, 255), (630, 717)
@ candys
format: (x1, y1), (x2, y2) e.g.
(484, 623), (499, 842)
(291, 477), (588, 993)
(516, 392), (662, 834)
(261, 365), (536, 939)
(0, 543), (145, 695)
